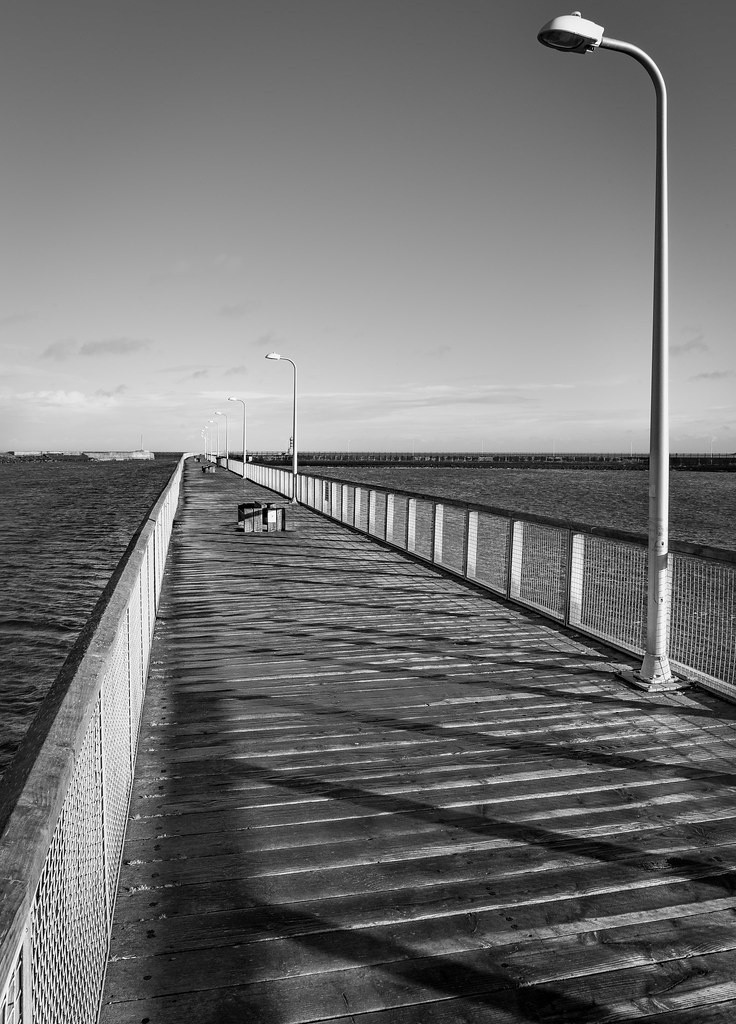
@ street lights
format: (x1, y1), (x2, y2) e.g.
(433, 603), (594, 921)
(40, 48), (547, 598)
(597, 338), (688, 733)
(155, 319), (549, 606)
(228, 397), (248, 479)
(200, 426), (213, 463)
(215, 411), (229, 471)
(266, 353), (306, 504)
(538, 10), (693, 689)
